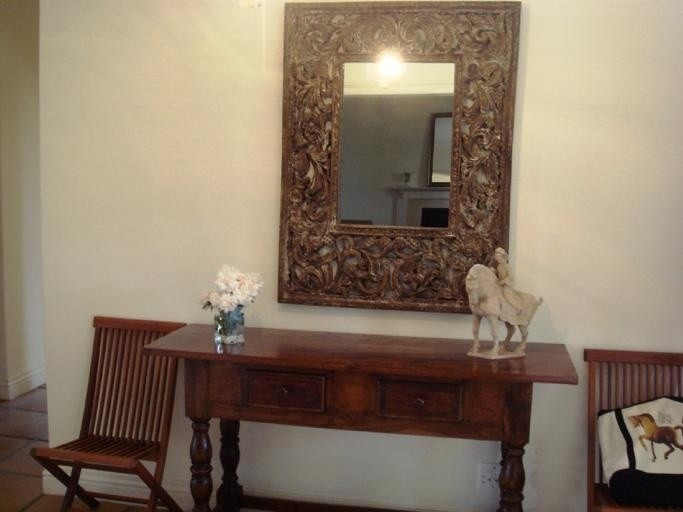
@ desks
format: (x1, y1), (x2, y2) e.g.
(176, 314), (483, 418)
(142, 322), (580, 512)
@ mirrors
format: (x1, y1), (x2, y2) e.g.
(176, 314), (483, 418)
(327, 53), (464, 239)
(427, 109), (454, 190)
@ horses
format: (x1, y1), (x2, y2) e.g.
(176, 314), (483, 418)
(626, 413), (683, 462)
(465, 264), (543, 357)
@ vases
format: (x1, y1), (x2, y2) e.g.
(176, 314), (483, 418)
(211, 314), (248, 347)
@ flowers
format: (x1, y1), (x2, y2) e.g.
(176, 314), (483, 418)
(197, 262), (265, 315)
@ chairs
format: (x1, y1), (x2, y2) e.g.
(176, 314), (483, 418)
(29, 313), (188, 512)
(582, 348), (683, 512)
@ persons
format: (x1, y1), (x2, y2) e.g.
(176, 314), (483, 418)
(492, 246), (523, 317)
